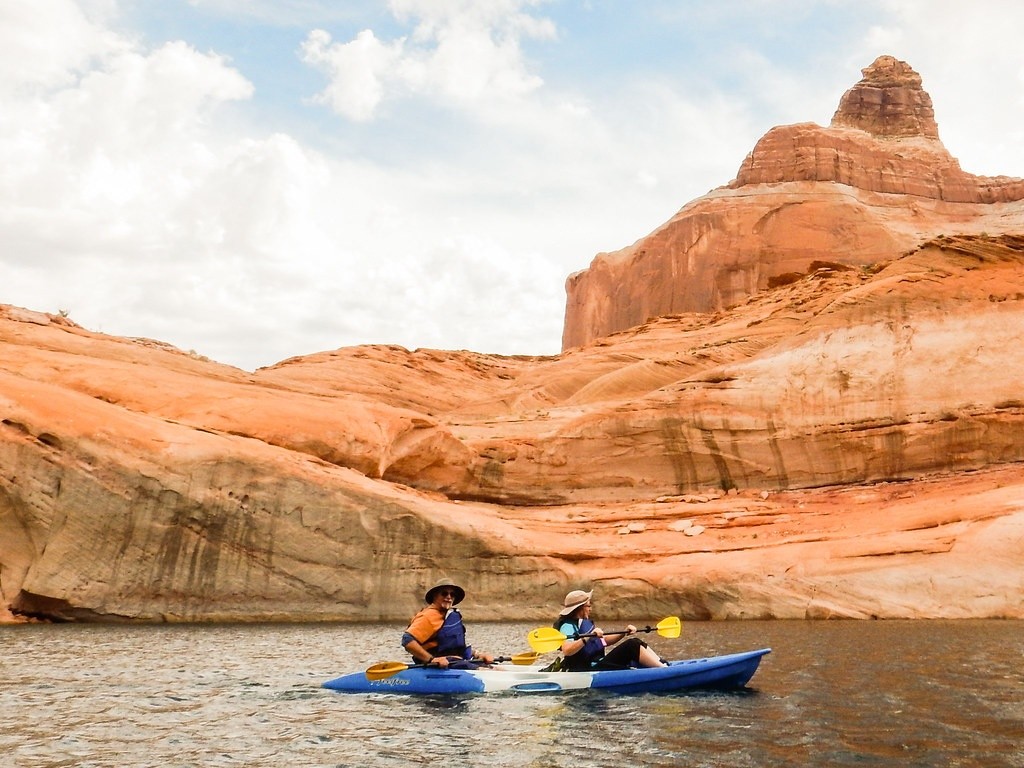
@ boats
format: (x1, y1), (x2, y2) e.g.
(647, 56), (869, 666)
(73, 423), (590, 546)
(318, 646), (773, 701)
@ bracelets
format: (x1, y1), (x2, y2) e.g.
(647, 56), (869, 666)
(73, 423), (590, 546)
(427, 657), (434, 663)
(582, 638), (587, 645)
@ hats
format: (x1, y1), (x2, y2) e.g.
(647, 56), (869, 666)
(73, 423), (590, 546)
(425, 578), (466, 606)
(559, 589), (594, 615)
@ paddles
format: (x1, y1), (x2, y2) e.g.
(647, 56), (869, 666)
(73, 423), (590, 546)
(366, 651), (545, 680)
(527, 617), (681, 654)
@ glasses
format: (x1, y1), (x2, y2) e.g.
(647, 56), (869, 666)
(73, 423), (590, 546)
(584, 605), (591, 608)
(439, 590), (457, 597)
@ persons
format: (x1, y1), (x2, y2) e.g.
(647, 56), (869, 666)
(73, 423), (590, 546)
(402, 578), (495, 667)
(553, 590), (670, 671)
